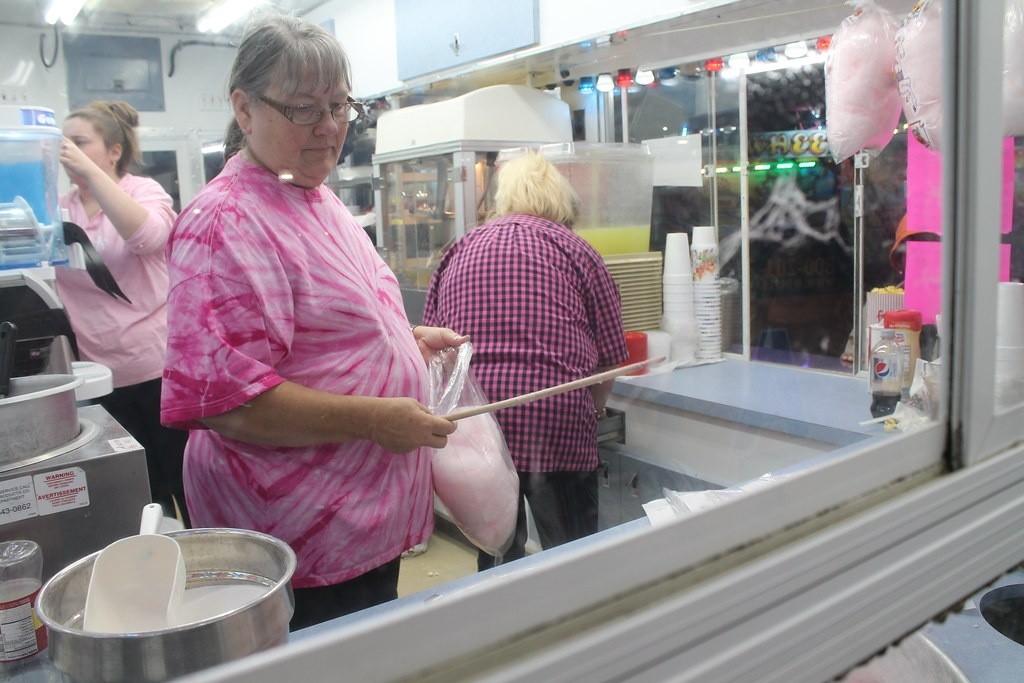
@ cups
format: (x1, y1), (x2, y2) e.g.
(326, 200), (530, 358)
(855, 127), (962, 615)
(646, 226), (722, 366)
(616, 331), (649, 377)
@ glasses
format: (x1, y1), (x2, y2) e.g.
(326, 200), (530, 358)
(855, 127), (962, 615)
(239, 85), (363, 126)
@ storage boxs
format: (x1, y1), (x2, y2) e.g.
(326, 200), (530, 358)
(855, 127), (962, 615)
(539, 141), (655, 257)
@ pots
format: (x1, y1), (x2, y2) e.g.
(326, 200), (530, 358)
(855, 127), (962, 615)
(30, 525), (297, 683)
(0, 372), (86, 465)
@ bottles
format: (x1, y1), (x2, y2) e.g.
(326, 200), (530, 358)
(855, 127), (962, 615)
(867, 329), (903, 424)
(0, 540), (62, 683)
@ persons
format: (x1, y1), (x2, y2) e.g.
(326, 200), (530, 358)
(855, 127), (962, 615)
(423, 152), (631, 572)
(161, 12), (470, 633)
(42, 103), (191, 529)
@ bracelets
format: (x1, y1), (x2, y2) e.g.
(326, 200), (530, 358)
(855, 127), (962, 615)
(410, 323), (417, 332)
